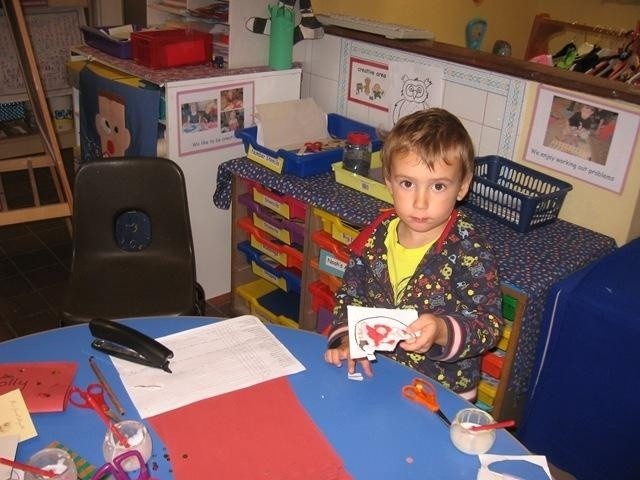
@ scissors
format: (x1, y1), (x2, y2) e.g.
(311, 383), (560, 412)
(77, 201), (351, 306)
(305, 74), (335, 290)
(402, 377), (451, 427)
(92, 451), (149, 480)
(68, 384), (122, 423)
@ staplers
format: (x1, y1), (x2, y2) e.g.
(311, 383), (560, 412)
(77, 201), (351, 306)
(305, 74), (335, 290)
(89, 319), (174, 374)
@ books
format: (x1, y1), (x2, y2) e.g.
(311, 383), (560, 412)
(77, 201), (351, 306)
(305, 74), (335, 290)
(210, 38), (230, 64)
(160, 0), (187, 9)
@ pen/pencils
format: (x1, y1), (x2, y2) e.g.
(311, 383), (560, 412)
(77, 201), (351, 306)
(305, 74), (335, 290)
(89, 357), (125, 416)
(468, 420), (515, 430)
(0, 458), (54, 478)
(86, 394), (130, 449)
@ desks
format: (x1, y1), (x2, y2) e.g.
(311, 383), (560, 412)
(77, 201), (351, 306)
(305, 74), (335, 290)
(0, 315), (550, 480)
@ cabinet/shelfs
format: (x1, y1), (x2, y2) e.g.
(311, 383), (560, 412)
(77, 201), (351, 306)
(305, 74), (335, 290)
(289, 36), (640, 250)
(67, 36), (301, 303)
(214, 112), (616, 424)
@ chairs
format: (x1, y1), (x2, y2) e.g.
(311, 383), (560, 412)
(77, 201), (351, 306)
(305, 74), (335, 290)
(64, 156), (206, 318)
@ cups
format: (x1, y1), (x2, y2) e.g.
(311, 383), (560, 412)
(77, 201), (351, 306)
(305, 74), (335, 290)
(101, 419), (154, 474)
(447, 407), (498, 456)
(23, 447), (79, 480)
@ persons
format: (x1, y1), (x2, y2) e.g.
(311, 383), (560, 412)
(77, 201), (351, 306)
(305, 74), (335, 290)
(323, 107), (506, 407)
(182, 87), (244, 133)
(563, 105), (596, 139)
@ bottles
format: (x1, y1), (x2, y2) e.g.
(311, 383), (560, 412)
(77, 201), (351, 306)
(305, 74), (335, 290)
(268, 2), (296, 71)
(341, 129), (373, 180)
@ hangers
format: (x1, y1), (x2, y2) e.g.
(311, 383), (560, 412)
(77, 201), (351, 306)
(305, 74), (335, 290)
(552, 20), (640, 83)
